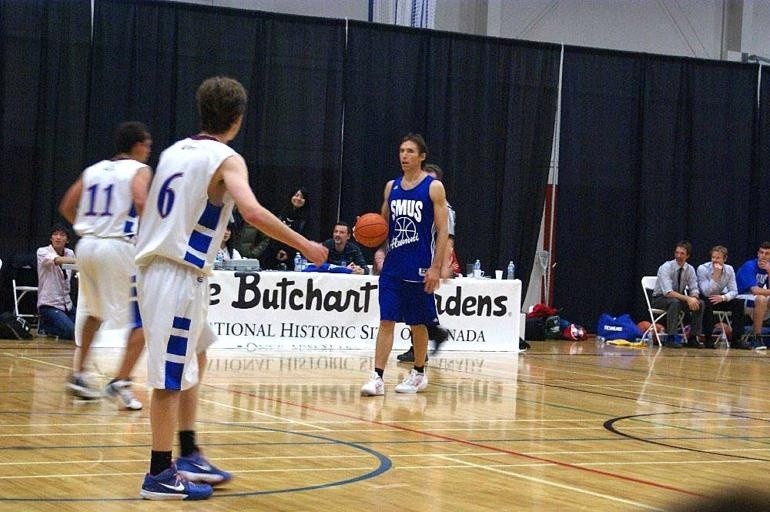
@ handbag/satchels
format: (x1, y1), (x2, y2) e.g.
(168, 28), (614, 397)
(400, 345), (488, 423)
(597, 313), (643, 341)
(526, 304), (587, 341)
(0, 312), (32, 339)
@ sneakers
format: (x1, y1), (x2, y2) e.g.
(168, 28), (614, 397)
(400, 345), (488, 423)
(362, 372), (383, 396)
(63, 373), (98, 399)
(752, 335), (767, 349)
(397, 330), (447, 393)
(730, 340), (748, 349)
(177, 450), (229, 484)
(37, 319), (48, 337)
(689, 336), (699, 347)
(705, 337), (714, 348)
(104, 379), (142, 409)
(667, 335), (679, 347)
(141, 463), (213, 500)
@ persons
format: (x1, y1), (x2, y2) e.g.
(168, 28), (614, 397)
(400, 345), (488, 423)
(262, 185), (311, 269)
(396, 163), (457, 362)
(650, 240), (770, 350)
(352, 131), (449, 396)
(134, 74), (328, 501)
(215, 217), (243, 261)
(319, 220), (370, 275)
(35, 224), (75, 340)
(372, 240), (387, 275)
(57, 120), (153, 410)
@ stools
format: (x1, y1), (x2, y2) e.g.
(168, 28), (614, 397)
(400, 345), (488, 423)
(740, 315), (770, 348)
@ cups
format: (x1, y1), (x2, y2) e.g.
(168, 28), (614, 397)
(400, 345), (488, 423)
(226, 258), (260, 271)
(495, 270), (503, 280)
(473, 269), (484, 278)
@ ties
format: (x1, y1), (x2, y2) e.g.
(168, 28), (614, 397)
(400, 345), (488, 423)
(678, 269), (682, 292)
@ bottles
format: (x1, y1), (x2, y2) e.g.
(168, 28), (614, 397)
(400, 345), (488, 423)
(216, 252), (223, 269)
(507, 260), (515, 280)
(293, 252), (302, 272)
(473, 259), (480, 270)
(594, 336), (606, 342)
(647, 331), (653, 347)
(718, 333), (727, 350)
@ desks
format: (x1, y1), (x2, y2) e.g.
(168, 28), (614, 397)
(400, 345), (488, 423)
(75, 266), (522, 356)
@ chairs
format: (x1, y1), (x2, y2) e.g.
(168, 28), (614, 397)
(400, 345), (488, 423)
(682, 283), (738, 349)
(11, 265), (45, 333)
(640, 275), (689, 347)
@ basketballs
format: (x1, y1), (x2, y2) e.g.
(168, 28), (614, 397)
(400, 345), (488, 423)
(638, 321), (664, 344)
(714, 323), (731, 341)
(354, 214), (388, 246)
